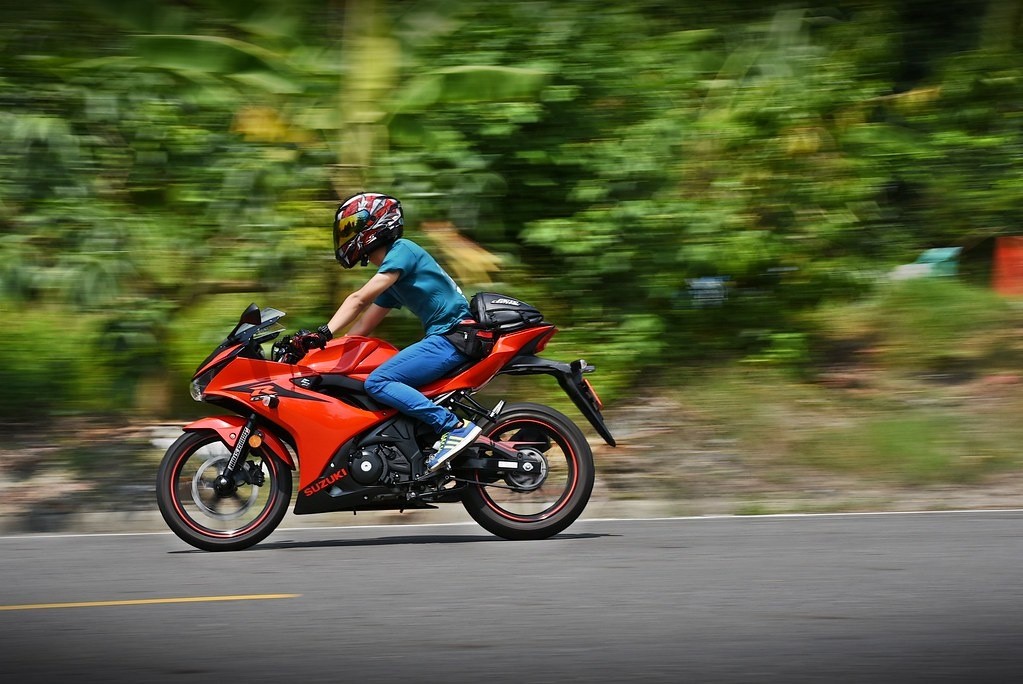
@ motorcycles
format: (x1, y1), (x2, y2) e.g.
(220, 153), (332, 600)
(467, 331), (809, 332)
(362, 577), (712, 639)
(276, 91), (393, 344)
(154, 289), (620, 553)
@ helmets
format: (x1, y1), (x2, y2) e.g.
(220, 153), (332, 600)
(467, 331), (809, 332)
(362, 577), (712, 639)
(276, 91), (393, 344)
(333, 191), (404, 270)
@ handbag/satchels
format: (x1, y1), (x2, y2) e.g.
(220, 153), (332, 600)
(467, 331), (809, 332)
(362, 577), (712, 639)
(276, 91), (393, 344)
(443, 324), (482, 361)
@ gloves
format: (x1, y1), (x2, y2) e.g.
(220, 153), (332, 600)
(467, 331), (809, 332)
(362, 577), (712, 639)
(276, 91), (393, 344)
(286, 328), (326, 359)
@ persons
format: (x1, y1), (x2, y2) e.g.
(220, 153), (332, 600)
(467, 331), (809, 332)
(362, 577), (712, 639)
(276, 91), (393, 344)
(291, 192), (482, 471)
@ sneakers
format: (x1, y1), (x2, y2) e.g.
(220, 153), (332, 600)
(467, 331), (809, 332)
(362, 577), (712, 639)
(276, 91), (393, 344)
(427, 418), (482, 470)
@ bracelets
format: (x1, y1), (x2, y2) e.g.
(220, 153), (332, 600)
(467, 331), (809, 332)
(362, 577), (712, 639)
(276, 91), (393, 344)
(318, 325), (333, 340)
